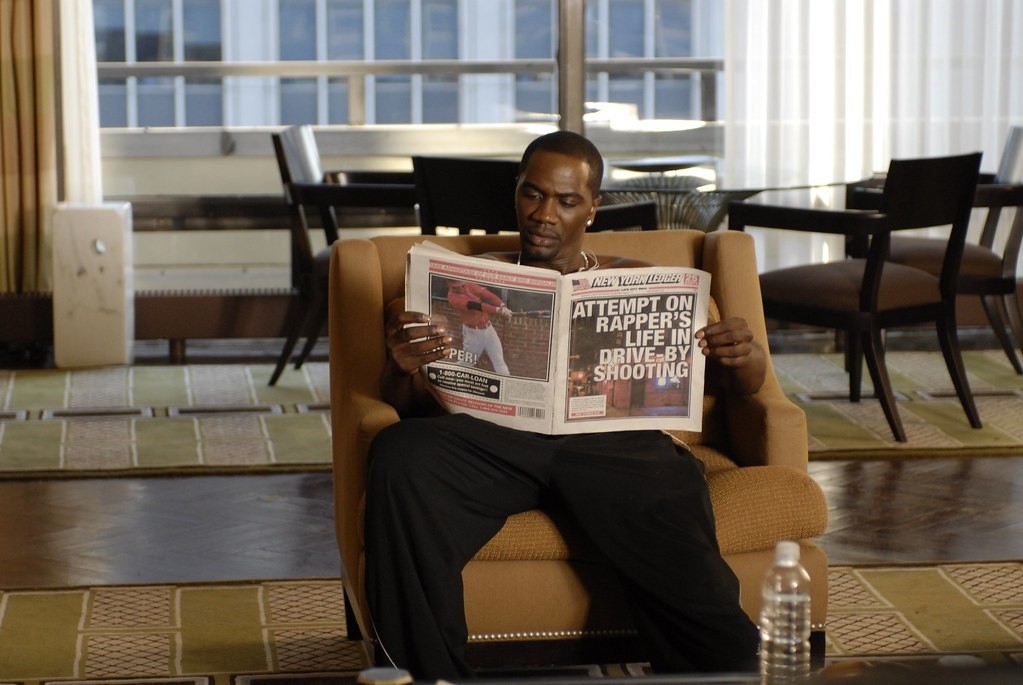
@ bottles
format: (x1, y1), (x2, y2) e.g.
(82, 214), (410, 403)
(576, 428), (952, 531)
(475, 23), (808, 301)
(760, 541), (811, 685)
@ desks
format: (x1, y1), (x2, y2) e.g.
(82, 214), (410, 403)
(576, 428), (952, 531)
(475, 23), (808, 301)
(600, 153), (874, 232)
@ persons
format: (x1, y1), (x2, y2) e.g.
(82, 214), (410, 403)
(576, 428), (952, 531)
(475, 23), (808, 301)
(444, 276), (513, 377)
(362, 132), (767, 685)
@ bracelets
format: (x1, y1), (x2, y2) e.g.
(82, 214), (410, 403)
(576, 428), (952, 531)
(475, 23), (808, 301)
(498, 307), (503, 315)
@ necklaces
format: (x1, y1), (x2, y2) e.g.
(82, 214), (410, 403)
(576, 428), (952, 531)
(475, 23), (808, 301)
(514, 248), (600, 273)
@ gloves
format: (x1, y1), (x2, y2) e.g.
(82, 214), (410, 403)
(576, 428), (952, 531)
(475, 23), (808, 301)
(495, 302), (512, 318)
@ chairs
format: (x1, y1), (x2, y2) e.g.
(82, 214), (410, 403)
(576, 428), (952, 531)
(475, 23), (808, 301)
(271, 122), (1023, 443)
(329, 230), (828, 676)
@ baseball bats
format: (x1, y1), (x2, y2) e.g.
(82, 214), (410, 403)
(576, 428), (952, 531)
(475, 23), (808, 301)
(511, 309), (551, 315)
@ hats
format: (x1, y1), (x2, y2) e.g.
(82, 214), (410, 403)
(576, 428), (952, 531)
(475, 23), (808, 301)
(447, 278), (466, 287)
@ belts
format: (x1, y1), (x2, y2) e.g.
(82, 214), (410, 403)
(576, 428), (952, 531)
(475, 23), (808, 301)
(465, 321), (490, 329)
